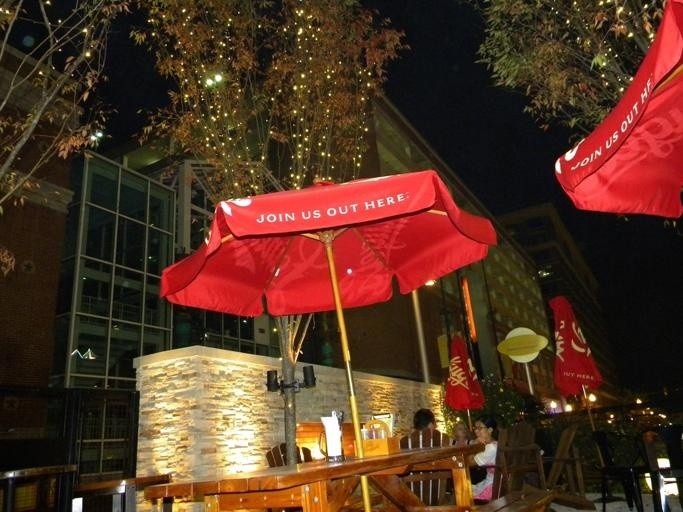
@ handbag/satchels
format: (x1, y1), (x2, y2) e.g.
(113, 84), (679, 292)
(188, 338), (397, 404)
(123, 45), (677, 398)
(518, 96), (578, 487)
(469, 466), (486, 483)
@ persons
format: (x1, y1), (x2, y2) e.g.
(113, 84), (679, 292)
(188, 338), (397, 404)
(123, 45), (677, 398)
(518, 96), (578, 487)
(398, 408), (458, 505)
(468, 418), (498, 505)
(444, 421), (476, 447)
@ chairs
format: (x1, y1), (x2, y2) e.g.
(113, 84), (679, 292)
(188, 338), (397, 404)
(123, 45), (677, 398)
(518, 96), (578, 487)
(266, 442), (312, 467)
(473, 420), (683, 512)
(398, 430), (451, 506)
(0, 463), (171, 512)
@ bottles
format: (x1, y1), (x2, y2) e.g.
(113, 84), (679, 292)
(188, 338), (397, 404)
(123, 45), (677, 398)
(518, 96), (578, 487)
(361, 424), (388, 440)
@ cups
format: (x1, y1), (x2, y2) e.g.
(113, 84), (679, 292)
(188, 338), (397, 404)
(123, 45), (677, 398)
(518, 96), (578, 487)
(320, 416), (344, 463)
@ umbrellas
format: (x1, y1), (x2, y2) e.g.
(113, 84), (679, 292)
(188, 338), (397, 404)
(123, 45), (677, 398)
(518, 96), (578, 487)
(445, 327), (486, 431)
(159, 168), (498, 508)
(547, 295), (604, 464)
(553, 0), (683, 216)
